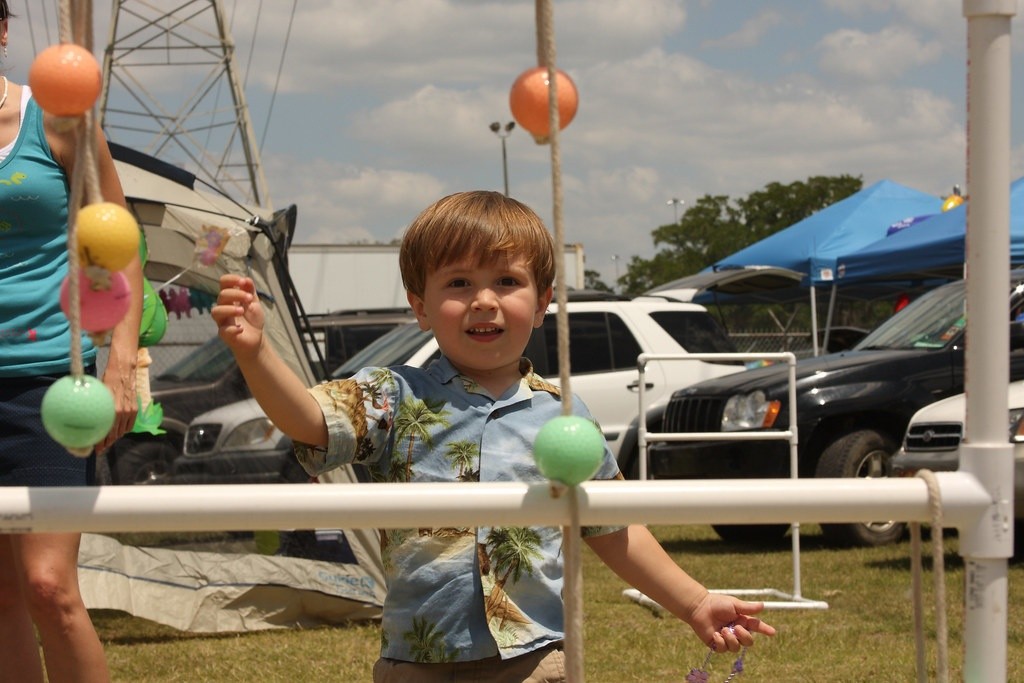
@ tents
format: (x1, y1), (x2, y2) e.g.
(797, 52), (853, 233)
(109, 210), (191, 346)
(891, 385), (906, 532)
(78, 141), (388, 636)
(690, 175), (1024, 359)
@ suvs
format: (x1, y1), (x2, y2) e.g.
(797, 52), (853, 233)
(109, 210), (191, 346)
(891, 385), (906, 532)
(94, 306), (419, 485)
(648, 262), (1024, 550)
(166, 265), (806, 558)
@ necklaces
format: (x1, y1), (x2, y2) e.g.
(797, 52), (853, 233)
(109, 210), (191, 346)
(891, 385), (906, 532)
(0, 76), (8, 108)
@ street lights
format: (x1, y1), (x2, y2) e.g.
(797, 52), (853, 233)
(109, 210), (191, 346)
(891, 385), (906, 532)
(490, 120), (515, 199)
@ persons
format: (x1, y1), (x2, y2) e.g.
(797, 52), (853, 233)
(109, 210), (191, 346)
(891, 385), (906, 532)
(212, 190), (776, 683)
(0, 0), (144, 683)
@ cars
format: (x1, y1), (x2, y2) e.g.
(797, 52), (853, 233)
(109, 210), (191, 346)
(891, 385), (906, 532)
(892, 381), (1024, 568)
